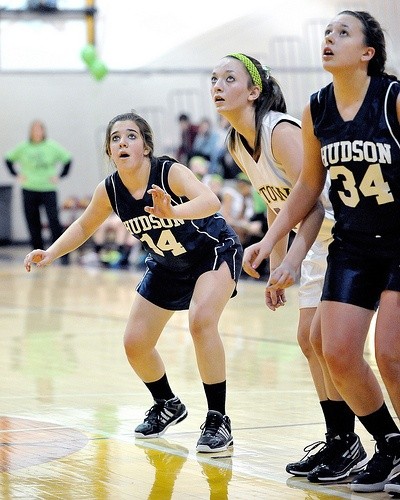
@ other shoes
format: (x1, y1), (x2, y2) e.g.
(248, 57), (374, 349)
(112, 251), (149, 270)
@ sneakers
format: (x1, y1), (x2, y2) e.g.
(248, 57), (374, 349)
(352, 452), (400, 491)
(285, 447), (326, 476)
(134, 396), (188, 439)
(197, 410), (234, 453)
(306, 435), (368, 483)
(385, 474), (400, 493)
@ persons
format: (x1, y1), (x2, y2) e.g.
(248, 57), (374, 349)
(23, 111), (240, 453)
(93, 115), (276, 276)
(5, 118), (73, 264)
(209, 52), (368, 484)
(242, 11), (400, 495)
